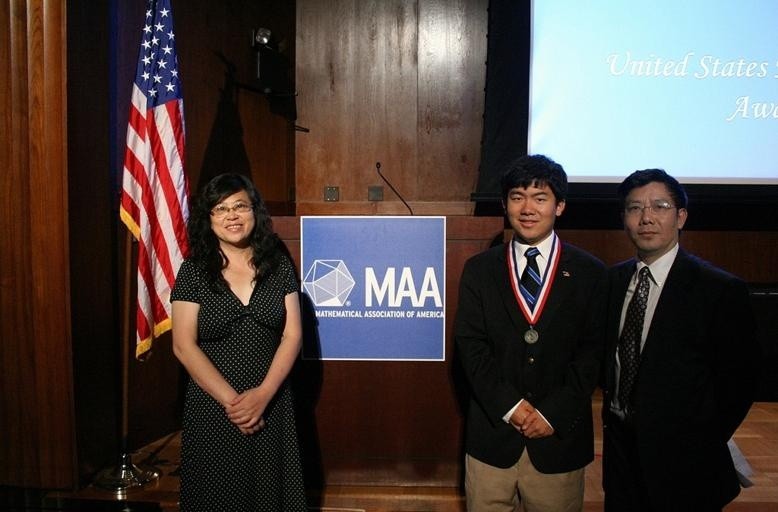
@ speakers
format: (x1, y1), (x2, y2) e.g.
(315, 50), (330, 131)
(268, 52), (296, 114)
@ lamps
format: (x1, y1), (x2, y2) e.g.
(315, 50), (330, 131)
(252, 29), (295, 99)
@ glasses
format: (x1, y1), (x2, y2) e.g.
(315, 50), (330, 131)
(208, 202), (253, 217)
(624, 200), (675, 215)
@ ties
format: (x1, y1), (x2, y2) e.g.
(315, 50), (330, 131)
(618, 265), (655, 406)
(518, 248), (541, 311)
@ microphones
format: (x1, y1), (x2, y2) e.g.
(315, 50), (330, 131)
(376, 162), (412, 216)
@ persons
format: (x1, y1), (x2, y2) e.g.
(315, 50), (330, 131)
(599, 167), (757, 512)
(170, 171), (311, 510)
(448, 152), (612, 511)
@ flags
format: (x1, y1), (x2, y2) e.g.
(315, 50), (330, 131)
(117, 0), (196, 364)
